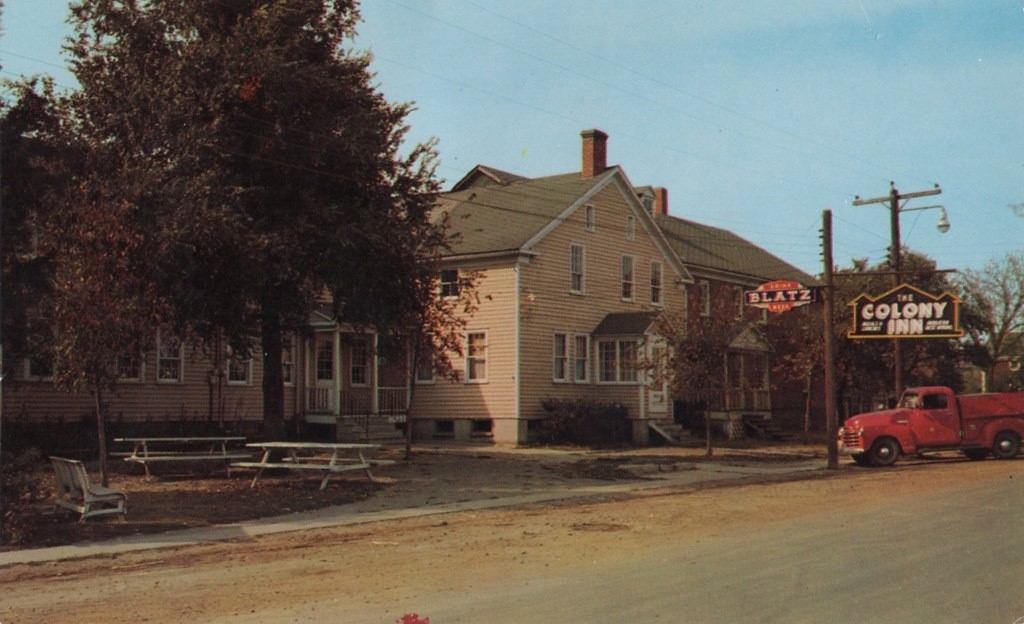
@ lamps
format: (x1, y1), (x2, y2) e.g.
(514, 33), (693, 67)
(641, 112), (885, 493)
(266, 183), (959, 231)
(522, 287), (538, 301)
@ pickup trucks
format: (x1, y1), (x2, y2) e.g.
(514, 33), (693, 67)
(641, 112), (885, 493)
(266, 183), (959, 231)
(841, 385), (1024, 467)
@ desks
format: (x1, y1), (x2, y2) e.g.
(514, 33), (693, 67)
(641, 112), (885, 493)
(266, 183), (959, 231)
(244, 441), (383, 491)
(113, 436), (247, 482)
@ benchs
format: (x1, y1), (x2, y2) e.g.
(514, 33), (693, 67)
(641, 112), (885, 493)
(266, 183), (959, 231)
(108, 451), (254, 463)
(49, 455), (129, 524)
(229, 457), (397, 471)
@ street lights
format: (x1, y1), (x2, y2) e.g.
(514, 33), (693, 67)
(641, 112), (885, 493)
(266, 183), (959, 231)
(890, 203), (951, 406)
